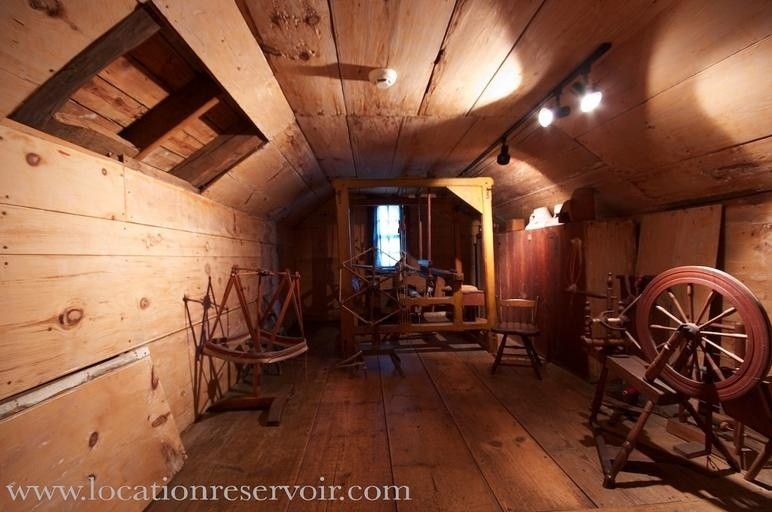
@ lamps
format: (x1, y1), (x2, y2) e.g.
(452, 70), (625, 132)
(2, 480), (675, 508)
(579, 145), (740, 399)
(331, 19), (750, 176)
(497, 139), (510, 165)
(539, 65), (601, 127)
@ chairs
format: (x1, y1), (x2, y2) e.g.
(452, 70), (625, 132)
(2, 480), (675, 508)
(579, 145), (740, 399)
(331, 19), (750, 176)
(491, 295), (545, 381)
